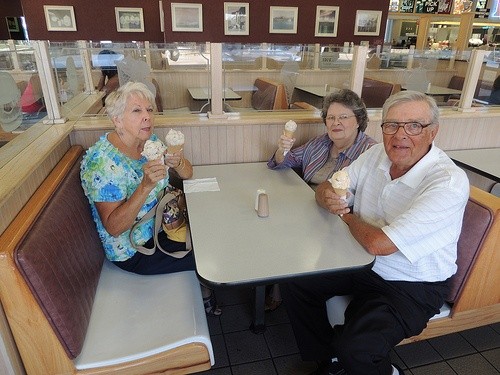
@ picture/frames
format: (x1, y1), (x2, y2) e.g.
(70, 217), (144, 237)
(44, 5), (77, 32)
(223, 2), (250, 36)
(354, 10), (383, 36)
(314, 5), (339, 37)
(269, 6), (299, 34)
(114, 6), (145, 32)
(171, 3), (204, 32)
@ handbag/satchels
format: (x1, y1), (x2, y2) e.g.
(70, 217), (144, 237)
(125, 186), (192, 258)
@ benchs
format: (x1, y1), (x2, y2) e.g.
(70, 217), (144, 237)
(225, 78), (289, 112)
(0, 146), (216, 375)
(151, 78), (191, 114)
(435, 76), (483, 107)
(325, 158), (500, 347)
(361, 77), (401, 107)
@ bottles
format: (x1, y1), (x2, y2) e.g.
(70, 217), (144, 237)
(258, 194), (269, 217)
(254, 189), (267, 212)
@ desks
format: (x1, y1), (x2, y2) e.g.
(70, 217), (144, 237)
(443, 147), (500, 183)
(182, 161), (376, 336)
(189, 88), (241, 111)
(295, 86), (339, 108)
(401, 84), (462, 102)
(0, 111), (47, 142)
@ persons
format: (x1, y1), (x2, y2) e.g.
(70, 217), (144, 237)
(120, 14), (140, 28)
(267, 88), (378, 311)
(50, 13), (71, 27)
(80, 82), (226, 314)
(315, 90), (470, 375)
(98, 49), (163, 114)
(21, 73), (64, 113)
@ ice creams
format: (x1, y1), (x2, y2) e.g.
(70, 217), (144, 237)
(141, 140), (166, 162)
(331, 170), (351, 199)
(285, 120), (297, 137)
(165, 128), (185, 156)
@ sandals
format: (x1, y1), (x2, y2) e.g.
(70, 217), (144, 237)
(203, 292), (225, 318)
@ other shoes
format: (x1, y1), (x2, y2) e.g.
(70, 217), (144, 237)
(313, 360), (346, 375)
(267, 295), (282, 312)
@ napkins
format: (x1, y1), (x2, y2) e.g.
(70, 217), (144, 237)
(182, 177), (220, 193)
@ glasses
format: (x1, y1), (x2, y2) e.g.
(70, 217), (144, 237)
(380, 121), (434, 136)
(324, 114), (355, 122)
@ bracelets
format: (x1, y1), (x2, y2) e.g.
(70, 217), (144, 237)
(174, 158), (185, 172)
